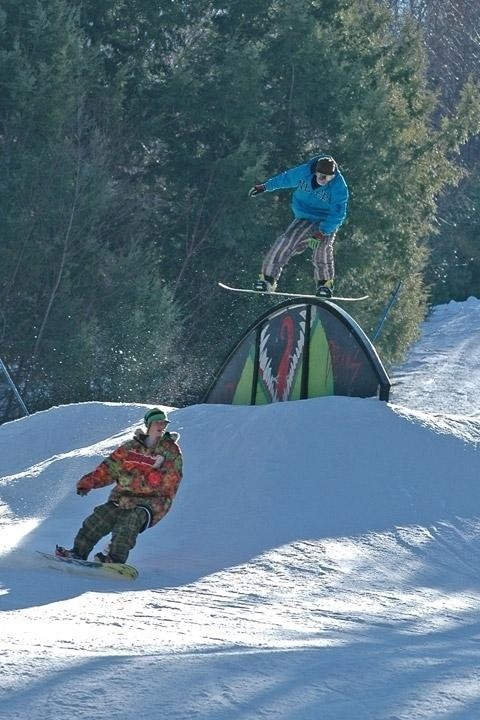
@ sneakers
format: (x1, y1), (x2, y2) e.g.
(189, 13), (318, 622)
(55, 544), (82, 560)
(94, 552), (116, 564)
(251, 273), (278, 292)
(316, 278), (334, 297)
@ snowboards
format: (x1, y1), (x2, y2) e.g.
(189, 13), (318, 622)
(36, 551), (140, 581)
(218, 280), (369, 301)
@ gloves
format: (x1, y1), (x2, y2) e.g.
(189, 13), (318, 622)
(309, 231), (326, 250)
(248, 184), (266, 199)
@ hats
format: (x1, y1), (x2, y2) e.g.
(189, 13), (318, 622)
(315, 157), (336, 175)
(144, 407), (171, 427)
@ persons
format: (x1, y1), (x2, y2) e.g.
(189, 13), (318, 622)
(246, 153), (351, 299)
(56, 408), (184, 564)
